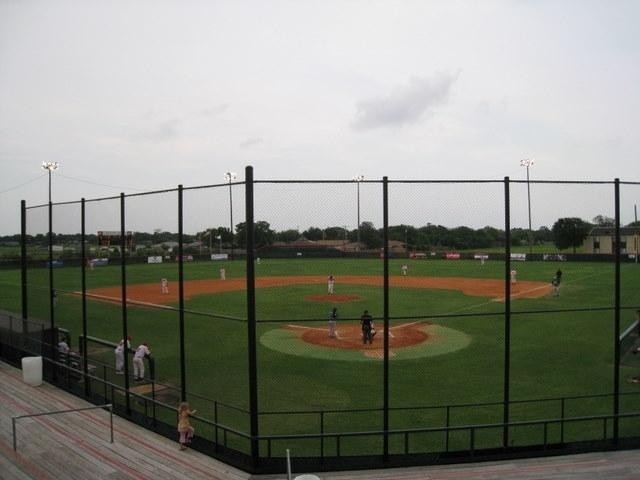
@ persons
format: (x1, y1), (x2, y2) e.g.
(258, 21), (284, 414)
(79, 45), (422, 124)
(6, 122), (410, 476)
(511, 268), (517, 285)
(58, 337), (70, 364)
(133, 341), (153, 380)
(402, 263), (408, 275)
(51, 286), (57, 307)
(328, 275), (335, 296)
(220, 265), (226, 280)
(361, 323), (376, 339)
(550, 276), (560, 297)
(257, 256), (261, 264)
(178, 402), (198, 451)
(115, 335), (134, 376)
(328, 307), (338, 336)
(555, 267), (563, 284)
(360, 310), (373, 344)
(89, 261), (94, 271)
(160, 274), (170, 294)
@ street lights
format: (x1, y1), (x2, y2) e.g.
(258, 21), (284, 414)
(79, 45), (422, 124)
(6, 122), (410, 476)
(224, 172), (236, 259)
(521, 159), (534, 261)
(42, 161), (59, 202)
(353, 176), (364, 252)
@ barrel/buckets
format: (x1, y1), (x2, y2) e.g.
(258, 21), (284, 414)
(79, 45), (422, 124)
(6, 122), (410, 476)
(21, 356), (43, 387)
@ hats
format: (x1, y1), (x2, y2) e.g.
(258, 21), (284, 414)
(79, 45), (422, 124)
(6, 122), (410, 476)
(127, 336), (132, 340)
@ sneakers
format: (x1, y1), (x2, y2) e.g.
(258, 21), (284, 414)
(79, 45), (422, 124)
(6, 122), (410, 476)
(133, 378), (144, 383)
(180, 439), (191, 450)
(115, 371), (124, 375)
